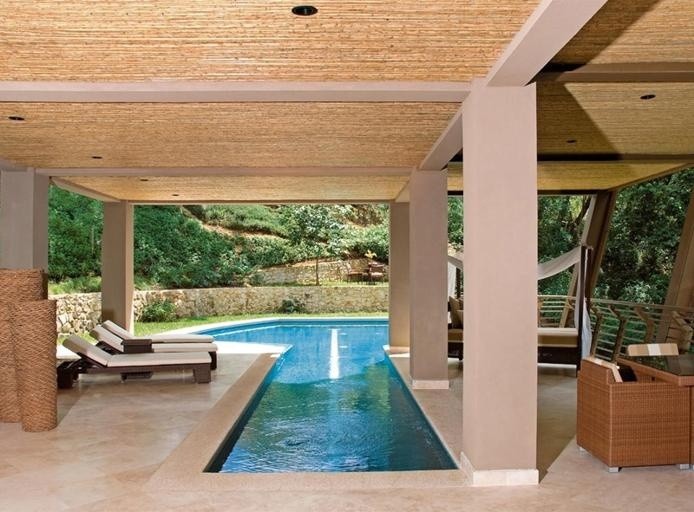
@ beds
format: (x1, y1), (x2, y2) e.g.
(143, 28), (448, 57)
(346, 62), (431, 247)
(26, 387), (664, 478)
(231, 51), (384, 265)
(447, 296), (583, 371)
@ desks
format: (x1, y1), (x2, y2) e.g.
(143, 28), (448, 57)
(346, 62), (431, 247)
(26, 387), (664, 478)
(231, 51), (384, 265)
(613, 355), (694, 387)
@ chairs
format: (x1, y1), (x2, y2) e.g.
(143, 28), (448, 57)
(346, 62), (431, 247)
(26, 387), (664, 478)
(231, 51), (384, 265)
(346, 260), (384, 285)
(576, 356), (691, 472)
(101, 319), (213, 342)
(627, 343), (679, 356)
(89, 325), (218, 370)
(56, 334), (213, 389)
